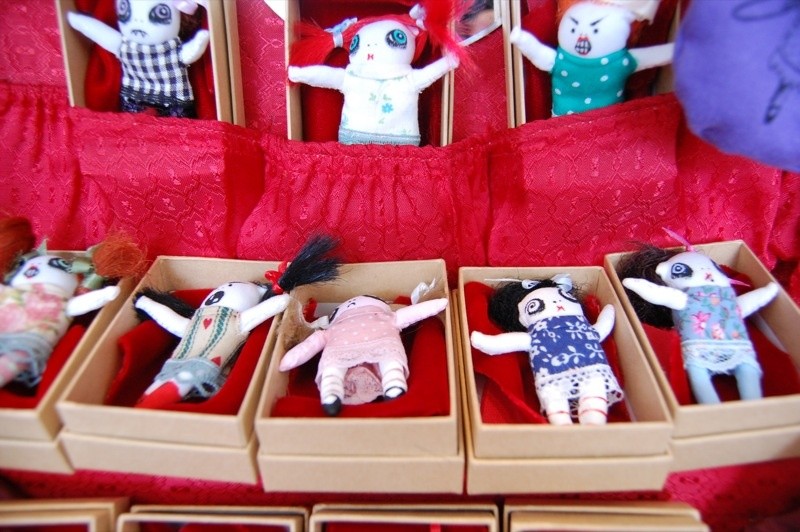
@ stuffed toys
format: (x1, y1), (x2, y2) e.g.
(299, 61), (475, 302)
(470, 273), (626, 424)
(616, 226), (780, 403)
(508, 0), (674, 120)
(288, 0), (460, 145)
(280, 277), (448, 417)
(67, 0), (211, 121)
(133, 233), (347, 410)
(0, 214), (155, 387)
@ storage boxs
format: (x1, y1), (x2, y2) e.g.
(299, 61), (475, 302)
(55, 0), (683, 147)
(0, 240), (800, 532)
